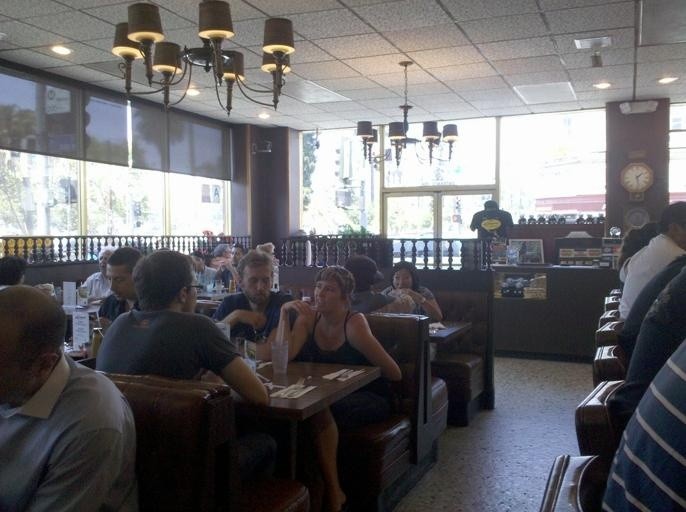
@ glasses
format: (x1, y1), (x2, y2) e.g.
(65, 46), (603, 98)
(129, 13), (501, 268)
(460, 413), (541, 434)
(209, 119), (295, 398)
(185, 283), (204, 291)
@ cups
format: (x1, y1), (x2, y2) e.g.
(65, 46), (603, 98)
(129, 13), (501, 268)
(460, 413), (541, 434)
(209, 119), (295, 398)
(271, 339), (289, 376)
(215, 279), (223, 295)
(229, 279), (237, 294)
(206, 280), (213, 293)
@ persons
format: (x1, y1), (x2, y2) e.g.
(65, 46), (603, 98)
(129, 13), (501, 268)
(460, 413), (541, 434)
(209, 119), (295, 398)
(0, 286), (138, 512)
(211, 251), (298, 359)
(345, 255), (416, 315)
(614, 253), (685, 371)
(619, 222), (662, 263)
(619, 202), (685, 320)
(79, 249), (116, 302)
(211, 244), (231, 275)
(470, 201), (513, 244)
(604, 337), (686, 511)
(214, 243), (248, 292)
(97, 247), (142, 336)
(189, 252), (218, 290)
(256, 242), (279, 292)
(380, 261), (443, 361)
(616, 267), (685, 423)
(273, 265), (402, 511)
(96, 250), (270, 512)
(0, 255), (26, 286)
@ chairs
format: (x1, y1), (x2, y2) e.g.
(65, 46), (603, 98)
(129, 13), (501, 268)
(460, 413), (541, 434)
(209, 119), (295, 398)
(540, 452), (608, 512)
(593, 344), (628, 390)
(604, 295), (621, 314)
(576, 381), (637, 456)
(593, 321), (627, 348)
(598, 309), (620, 330)
(608, 288), (622, 296)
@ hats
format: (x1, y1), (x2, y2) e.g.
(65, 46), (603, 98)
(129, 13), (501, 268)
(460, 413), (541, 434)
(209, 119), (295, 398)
(344, 255), (385, 280)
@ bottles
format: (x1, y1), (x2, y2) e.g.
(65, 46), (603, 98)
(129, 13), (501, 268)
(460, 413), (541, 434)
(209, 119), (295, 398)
(519, 216), (526, 225)
(547, 215), (558, 225)
(92, 328), (102, 357)
(229, 280), (236, 294)
(558, 216), (566, 225)
(528, 215), (536, 225)
(537, 215), (545, 225)
(597, 214), (606, 225)
(586, 215), (595, 226)
(576, 215), (585, 225)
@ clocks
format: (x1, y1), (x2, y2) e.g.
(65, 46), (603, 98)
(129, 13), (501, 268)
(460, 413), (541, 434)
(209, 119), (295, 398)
(621, 164), (655, 193)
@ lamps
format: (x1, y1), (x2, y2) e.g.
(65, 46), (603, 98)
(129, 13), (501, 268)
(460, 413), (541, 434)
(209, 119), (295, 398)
(112, 0), (295, 117)
(357, 58), (459, 164)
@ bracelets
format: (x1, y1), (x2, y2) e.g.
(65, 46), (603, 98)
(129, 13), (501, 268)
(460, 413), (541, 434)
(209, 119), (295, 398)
(420, 297), (426, 308)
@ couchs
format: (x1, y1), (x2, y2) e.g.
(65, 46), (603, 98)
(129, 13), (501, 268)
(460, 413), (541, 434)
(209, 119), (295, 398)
(351, 310), (449, 471)
(111, 380), (219, 512)
(325, 314), (424, 506)
(101, 370), (308, 512)
(426, 288), (489, 425)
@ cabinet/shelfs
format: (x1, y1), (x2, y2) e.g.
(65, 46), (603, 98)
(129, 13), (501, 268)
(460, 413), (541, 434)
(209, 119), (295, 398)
(492, 260), (622, 362)
(508, 222), (605, 263)
